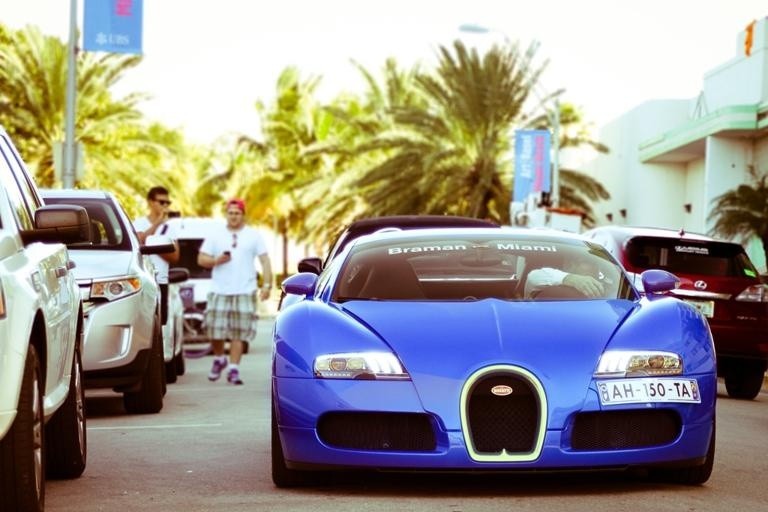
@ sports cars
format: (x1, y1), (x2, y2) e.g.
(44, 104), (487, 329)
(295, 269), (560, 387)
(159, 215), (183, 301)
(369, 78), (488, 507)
(269, 225), (721, 487)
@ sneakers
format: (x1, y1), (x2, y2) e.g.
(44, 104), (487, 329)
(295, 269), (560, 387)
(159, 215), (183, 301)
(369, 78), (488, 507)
(208, 357), (227, 381)
(227, 369), (242, 384)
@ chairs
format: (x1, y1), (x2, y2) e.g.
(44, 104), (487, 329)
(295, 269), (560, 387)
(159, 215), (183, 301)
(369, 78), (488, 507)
(353, 259), (428, 301)
(517, 252), (566, 298)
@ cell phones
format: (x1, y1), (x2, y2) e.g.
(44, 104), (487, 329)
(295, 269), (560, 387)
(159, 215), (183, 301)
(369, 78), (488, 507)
(168, 212), (180, 217)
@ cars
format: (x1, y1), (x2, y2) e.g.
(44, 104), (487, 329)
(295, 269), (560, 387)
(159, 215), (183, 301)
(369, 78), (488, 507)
(297, 216), (494, 275)
(577, 225), (767, 401)
(30, 186), (225, 416)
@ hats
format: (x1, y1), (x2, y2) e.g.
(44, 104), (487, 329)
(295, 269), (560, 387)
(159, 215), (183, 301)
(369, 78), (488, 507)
(225, 198), (245, 213)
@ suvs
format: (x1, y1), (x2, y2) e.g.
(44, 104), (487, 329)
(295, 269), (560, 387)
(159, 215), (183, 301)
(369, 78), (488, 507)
(1, 124), (91, 512)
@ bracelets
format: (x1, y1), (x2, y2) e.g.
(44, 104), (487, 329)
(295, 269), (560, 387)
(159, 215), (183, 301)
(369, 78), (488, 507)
(263, 282), (271, 289)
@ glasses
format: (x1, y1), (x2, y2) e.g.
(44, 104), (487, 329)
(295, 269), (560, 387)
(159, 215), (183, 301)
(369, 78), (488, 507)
(153, 198), (171, 205)
(231, 234), (237, 248)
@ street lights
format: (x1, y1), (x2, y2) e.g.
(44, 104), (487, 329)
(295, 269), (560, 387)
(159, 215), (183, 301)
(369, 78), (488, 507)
(456, 21), (565, 213)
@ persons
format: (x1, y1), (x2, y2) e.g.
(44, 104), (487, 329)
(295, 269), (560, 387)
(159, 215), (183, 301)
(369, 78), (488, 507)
(131, 186), (179, 327)
(196, 195), (274, 387)
(522, 251), (608, 300)
